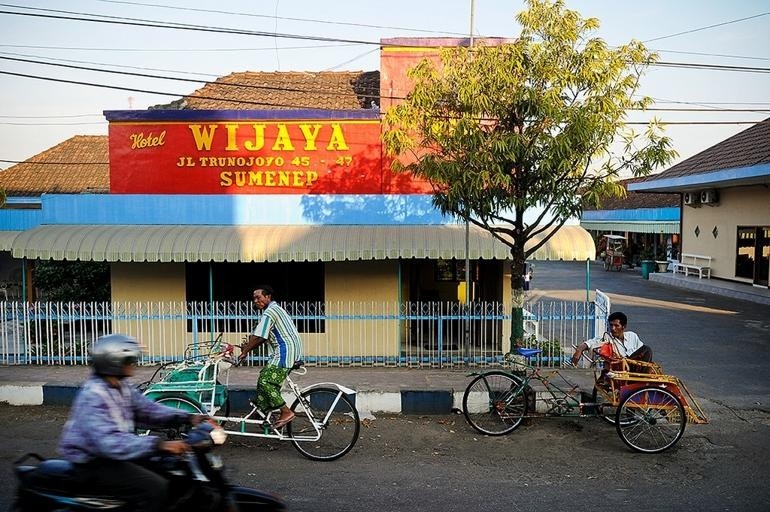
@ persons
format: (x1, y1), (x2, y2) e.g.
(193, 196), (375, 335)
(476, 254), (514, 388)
(572, 310), (653, 424)
(236, 284), (304, 448)
(55, 332), (217, 511)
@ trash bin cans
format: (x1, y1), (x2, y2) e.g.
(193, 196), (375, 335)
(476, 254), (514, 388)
(641, 260), (656, 280)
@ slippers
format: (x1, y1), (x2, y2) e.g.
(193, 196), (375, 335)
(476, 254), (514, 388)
(273, 414), (295, 428)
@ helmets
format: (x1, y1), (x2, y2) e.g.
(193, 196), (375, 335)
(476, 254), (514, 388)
(89, 335), (140, 378)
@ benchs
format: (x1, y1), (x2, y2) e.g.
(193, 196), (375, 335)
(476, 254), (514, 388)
(672, 253), (712, 280)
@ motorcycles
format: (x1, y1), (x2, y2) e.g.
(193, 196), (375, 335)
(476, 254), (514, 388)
(11, 409), (291, 512)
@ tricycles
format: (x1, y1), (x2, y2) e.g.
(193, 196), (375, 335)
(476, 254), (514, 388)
(139, 331), (362, 463)
(463, 343), (713, 455)
(601, 233), (626, 272)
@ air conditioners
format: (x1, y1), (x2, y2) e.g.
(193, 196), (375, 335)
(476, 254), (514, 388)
(683, 188), (720, 209)
